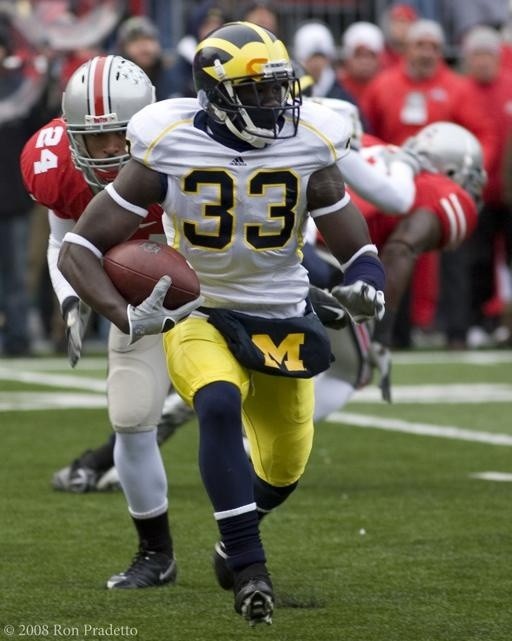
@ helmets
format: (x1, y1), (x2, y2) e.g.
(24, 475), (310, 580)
(59, 54), (158, 193)
(191, 20), (304, 149)
(408, 118), (489, 204)
(289, 59), (314, 98)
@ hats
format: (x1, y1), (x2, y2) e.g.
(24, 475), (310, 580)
(342, 21), (383, 60)
(294, 22), (335, 63)
(116, 13), (161, 51)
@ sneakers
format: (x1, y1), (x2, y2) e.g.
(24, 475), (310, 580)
(459, 322), (510, 352)
(105, 547), (177, 590)
(213, 538), (233, 590)
(54, 450), (122, 495)
(235, 567), (276, 629)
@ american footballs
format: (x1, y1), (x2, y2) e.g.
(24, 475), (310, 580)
(103, 240), (200, 309)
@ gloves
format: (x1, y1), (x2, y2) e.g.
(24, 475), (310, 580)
(355, 342), (394, 404)
(331, 282), (389, 326)
(122, 275), (206, 345)
(57, 296), (96, 367)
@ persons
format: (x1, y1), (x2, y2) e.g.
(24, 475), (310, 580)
(55, 16), (389, 626)
(48, 116), (492, 497)
(15, 48), (181, 591)
